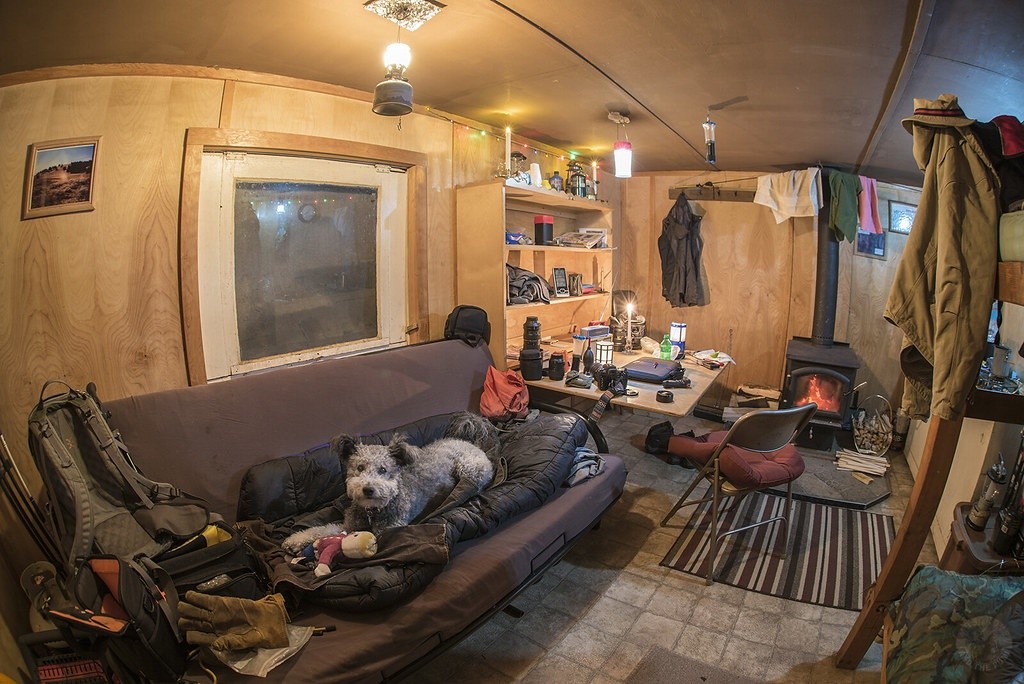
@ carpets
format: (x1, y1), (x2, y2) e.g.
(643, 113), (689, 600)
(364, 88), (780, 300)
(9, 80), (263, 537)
(660, 482), (897, 613)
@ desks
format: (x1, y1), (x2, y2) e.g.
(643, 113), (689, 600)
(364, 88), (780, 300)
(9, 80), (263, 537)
(938, 501), (1024, 578)
(510, 346), (732, 418)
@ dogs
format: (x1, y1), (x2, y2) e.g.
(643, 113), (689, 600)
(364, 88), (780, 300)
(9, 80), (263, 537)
(282, 411), (498, 553)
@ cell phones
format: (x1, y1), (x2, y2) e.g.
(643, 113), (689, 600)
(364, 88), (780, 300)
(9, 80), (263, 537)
(552, 267), (570, 298)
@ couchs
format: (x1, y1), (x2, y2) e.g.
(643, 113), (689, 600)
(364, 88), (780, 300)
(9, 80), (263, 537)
(56, 337), (629, 684)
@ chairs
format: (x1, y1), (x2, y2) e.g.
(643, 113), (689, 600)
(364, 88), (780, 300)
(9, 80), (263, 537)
(659, 402), (818, 586)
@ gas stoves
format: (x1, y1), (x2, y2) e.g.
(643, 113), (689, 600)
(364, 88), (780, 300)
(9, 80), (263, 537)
(975, 361), (1024, 395)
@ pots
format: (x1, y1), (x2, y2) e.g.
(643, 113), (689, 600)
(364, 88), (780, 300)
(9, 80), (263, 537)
(991, 345), (1012, 377)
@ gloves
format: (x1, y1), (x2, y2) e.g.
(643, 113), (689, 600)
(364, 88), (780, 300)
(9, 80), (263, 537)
(176, 588), (292, 651)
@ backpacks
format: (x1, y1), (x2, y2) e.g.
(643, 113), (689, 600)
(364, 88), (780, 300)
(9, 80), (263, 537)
(27, 379), (212, 577)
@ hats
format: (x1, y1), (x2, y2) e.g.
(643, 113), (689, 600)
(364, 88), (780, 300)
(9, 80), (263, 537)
(901, 93), (977, 136)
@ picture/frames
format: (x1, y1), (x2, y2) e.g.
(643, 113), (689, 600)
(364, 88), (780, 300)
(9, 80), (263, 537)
(852, 223), (888, 261)
(23, 135), (103, 219)
(887, 200), (918, 236)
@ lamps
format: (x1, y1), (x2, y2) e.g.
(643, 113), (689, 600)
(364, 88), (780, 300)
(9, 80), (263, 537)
(614, 121), (633, 178)
(363, 0), (444, 132)
(702, 111), (717, 163)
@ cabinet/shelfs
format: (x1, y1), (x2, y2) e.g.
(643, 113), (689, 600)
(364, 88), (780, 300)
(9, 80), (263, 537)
(455, 180), (612, 374)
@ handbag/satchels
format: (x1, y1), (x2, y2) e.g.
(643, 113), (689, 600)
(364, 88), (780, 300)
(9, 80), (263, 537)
(620, 356), (686, 384)
(154, 511), (274, 600)
(480, 364), (530, 424)
(47, 552), (191, 684)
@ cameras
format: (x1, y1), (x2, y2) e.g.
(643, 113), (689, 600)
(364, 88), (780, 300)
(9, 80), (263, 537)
(591, 363), (628, 391)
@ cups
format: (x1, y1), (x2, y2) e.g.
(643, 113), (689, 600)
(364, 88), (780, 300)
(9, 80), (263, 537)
(549, 354), (564, 380)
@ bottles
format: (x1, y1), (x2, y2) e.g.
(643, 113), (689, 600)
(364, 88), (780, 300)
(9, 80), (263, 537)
(993, 503), (1021, 556)
(549, 171), (563, 191)
(660, 334), (671, 360)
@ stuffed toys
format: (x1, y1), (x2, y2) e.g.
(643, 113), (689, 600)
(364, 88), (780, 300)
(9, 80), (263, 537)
(298, 528), (380, 580)
